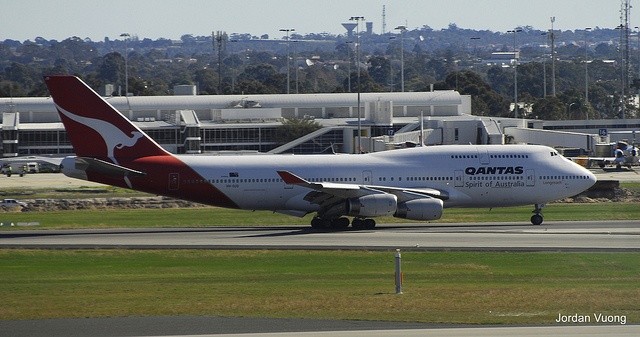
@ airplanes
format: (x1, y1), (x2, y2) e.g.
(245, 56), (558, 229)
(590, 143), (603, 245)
(568, 129), (640, 170)
(43, 73), (598, 231)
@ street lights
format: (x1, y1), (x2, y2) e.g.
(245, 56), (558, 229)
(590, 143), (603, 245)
(349, 15), (366, 153)
(541, 32), (546, 99)
(550, 31), (557, 97)
(120, 32), (130, 96)
(615, 24), (625, 118)
(395, 25), (407, 92)
(634, 25), (639, 71)
(278, 28), (294, 94)
(215, 35), (225, 94)
(344, 40), (353, 92)
(508, 29), (523, 118)
(585, 27), (591, 101)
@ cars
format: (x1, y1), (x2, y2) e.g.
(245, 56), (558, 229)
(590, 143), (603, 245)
(24, 162), (38, 173)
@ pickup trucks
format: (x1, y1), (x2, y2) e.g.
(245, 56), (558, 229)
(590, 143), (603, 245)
(0, 198), (25, 208)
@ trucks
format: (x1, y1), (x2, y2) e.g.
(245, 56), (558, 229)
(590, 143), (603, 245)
(0, 163), (23, 174)
